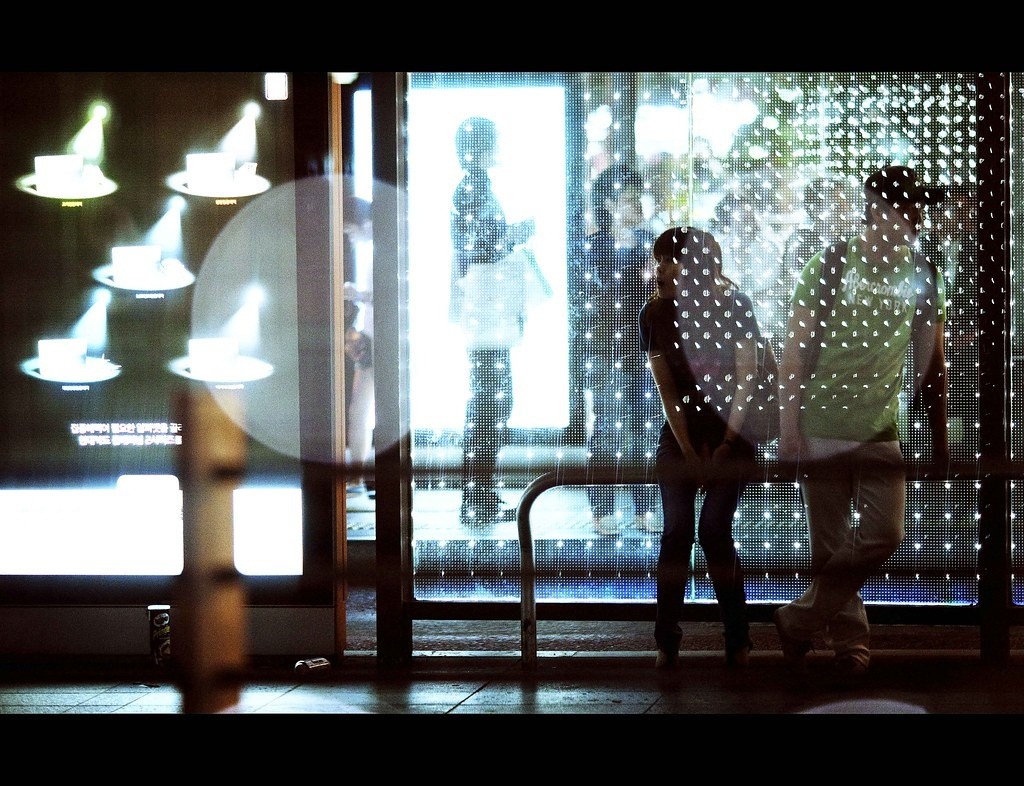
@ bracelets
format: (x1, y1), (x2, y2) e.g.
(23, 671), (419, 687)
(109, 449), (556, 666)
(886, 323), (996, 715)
(722, 439), (732, 445)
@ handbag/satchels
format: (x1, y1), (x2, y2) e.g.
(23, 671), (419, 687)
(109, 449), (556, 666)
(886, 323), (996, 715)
(725, 287), (782, 443)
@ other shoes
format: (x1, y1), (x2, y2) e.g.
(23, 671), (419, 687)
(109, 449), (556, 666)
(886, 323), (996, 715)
(833, 657), (866, 692)
(656, 647), (678, 668)
(774, 610), (818, 688)
(725, 648), (748, 669)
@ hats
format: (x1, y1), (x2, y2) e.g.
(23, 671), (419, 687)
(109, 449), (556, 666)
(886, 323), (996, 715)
(864, 165), (945, 206)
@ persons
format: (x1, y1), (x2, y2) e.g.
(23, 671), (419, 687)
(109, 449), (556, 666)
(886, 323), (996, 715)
(636, 227), (761, 671)
(917, 186), (978, 417)
(341, 196), (374, 497)
(771, 165), (951, 675)
(449, 116), (536, 525)
(781, 173), (849, 327)
(586, 164), (662, 535)
